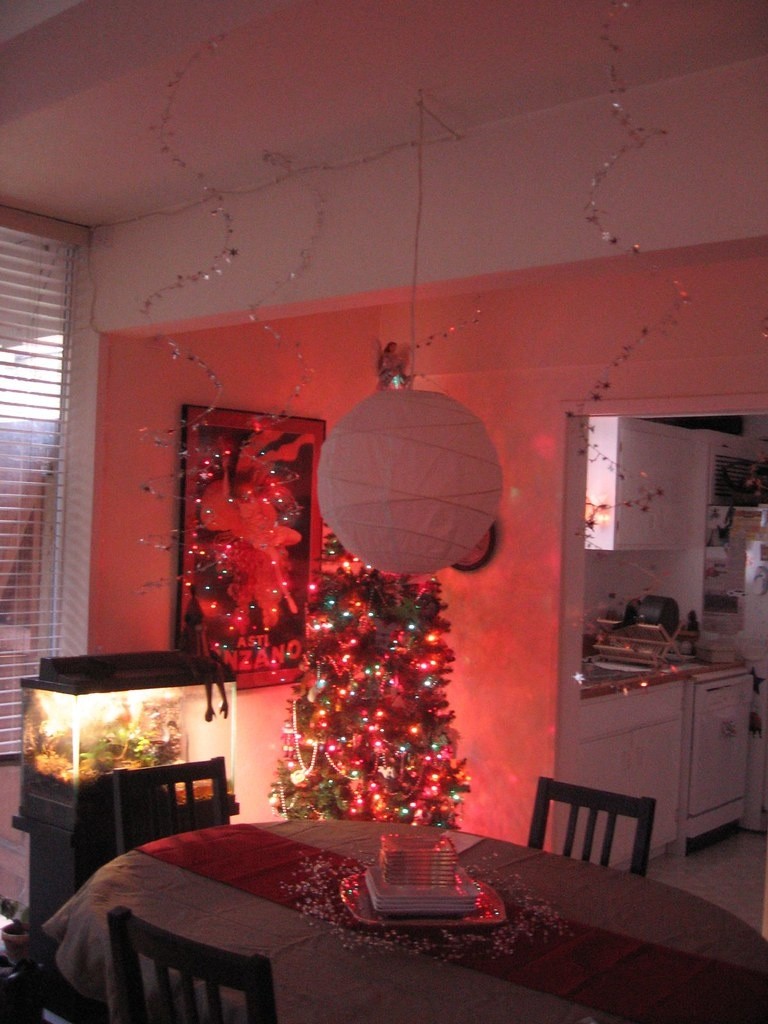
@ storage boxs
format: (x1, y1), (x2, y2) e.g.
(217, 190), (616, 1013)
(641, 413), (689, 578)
(17, 650), (237, 834)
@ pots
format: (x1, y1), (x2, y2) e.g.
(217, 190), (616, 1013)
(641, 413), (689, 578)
(611, 595), (678, 636)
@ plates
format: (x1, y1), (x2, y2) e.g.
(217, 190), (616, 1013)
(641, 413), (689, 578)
(340, 835), (506, 930)
(666, 654), (696, 663)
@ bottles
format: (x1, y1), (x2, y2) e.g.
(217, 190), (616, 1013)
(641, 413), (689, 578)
(687, 612), (698, 639)
(681, 635), (692, 655)
(607, 593), (616, 620)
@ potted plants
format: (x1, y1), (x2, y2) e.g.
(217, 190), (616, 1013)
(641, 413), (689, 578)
(0, 895), (30, 965)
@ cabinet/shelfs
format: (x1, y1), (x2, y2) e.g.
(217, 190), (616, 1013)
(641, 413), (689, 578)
(579, 680), (687, 872)
(580, 416), (694, 548)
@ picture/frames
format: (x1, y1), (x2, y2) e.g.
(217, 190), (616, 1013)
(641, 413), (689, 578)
(171, 405), (327, 693)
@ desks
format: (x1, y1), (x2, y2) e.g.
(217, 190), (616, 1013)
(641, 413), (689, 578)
(42, 820), (768, 1024)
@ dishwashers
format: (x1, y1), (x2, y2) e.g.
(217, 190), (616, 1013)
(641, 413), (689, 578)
(686, 667), (753, 838)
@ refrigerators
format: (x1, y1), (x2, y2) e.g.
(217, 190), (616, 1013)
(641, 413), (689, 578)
(704, 505), (768, 833)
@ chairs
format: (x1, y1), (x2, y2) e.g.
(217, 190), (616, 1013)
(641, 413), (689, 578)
(527, 777), (657, 877)
(106, 906), (279, 1024)
(114, 756), (231, 856)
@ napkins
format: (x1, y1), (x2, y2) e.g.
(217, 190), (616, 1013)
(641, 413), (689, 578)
(439, 830), (486, 854)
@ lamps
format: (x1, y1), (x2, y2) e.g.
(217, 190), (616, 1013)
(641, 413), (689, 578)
(319, 89), (500, 575)
